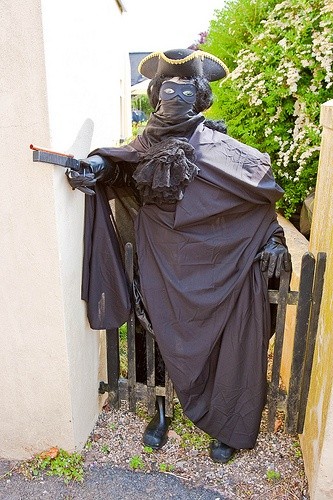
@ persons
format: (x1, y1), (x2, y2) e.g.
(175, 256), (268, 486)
(66, 47), (293, 463)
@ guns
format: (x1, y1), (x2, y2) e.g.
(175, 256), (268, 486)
(30, 145), (92, 178)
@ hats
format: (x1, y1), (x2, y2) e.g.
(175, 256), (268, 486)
(138, 49), (229, 82)
